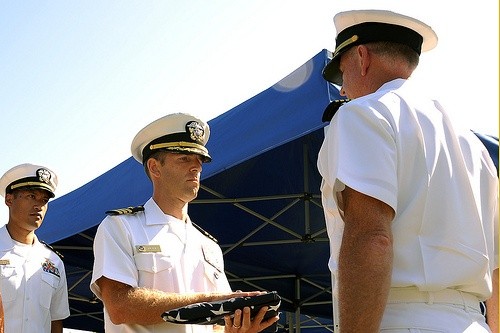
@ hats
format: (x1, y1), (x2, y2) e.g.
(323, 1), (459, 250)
(322, 9), (438, 86)
(0, 164), (57, 198)
(131, 113), (213, 166)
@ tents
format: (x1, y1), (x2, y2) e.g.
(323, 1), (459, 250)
(34, 49), (500, 333)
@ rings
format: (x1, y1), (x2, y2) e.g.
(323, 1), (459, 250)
(233, 324), (241, 328)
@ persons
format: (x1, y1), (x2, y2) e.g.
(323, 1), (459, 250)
(89, 113), (280, 333)
(0, 163), (71, 333)
(317, 10), (500, 333)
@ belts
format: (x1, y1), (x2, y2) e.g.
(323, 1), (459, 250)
(385, 287), (482, 312)
